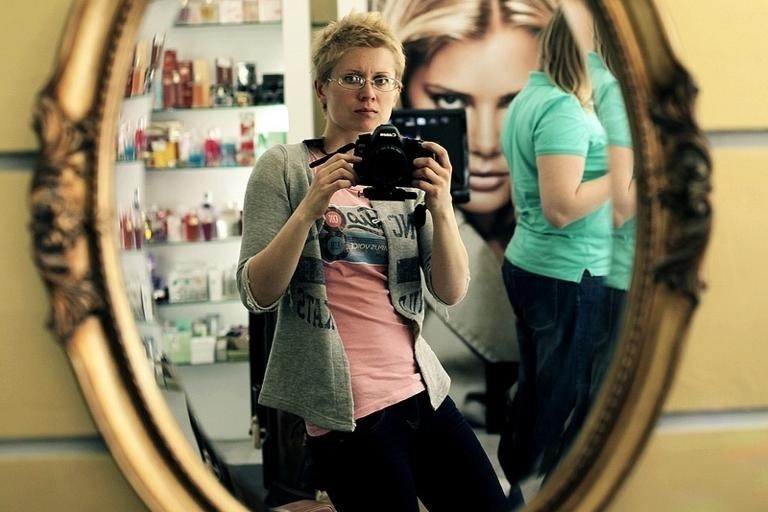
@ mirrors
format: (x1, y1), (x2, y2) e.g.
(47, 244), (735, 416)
(26, 0), (711, 512)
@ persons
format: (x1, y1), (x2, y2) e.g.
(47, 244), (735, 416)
(360, 3), (562, 430)
(569, 19), (641, 440)
(235, 9), (511, 512)
(474, 2), (618, 488)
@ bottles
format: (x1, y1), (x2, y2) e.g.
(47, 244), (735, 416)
(121, 186), (143, 250)
(145, 186), (217, 250)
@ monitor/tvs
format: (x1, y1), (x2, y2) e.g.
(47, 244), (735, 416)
(390, 108), (469, 203)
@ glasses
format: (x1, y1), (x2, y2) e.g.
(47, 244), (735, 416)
(327, 73), (403, 91)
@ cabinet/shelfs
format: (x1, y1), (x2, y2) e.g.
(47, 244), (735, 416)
(115, 95), (144, 326)
(144, 21), (290, 367)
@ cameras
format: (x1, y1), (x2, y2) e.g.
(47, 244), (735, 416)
(353, 123), (433, 200)
